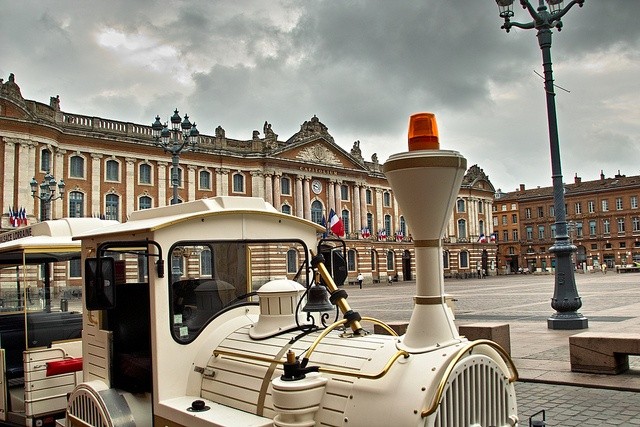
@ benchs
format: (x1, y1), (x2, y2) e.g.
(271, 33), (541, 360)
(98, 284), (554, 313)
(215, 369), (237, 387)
(569, 331), (640, 374)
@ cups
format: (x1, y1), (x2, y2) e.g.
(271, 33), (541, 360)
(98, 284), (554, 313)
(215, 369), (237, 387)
(60, 298), (68, 312)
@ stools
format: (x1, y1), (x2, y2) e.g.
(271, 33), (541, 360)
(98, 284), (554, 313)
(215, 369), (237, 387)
(460, 323), (511, 357)
(373, 322), (415, 338)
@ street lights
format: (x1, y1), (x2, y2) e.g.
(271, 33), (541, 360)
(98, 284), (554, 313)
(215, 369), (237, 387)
(30, 169), (65, 311)
(497, 1), (587, 329)
(152, 108), (199, 203)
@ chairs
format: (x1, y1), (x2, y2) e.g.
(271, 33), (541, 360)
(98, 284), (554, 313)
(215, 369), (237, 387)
(0, 311), (83, 375)
(112, 278), (224, 392)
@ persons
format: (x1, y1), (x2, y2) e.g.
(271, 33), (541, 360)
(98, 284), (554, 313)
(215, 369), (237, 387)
(387, 274), (393, 285)
(482, 268), (487, 278)
(477, 266), (481, 278)
(356, 273), (365, 288)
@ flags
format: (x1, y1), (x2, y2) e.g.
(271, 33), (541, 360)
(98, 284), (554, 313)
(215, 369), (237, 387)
(378, 229), (386, 238)
(395, 232), (403, 242)
(329, 207), (344, 236)
(490, 234), (496, 243)
(361, 227), (371, 238)
(480, 234), (486, 242)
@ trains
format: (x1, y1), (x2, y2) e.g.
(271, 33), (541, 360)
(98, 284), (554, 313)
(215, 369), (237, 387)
(1, 113), (549, 423)
